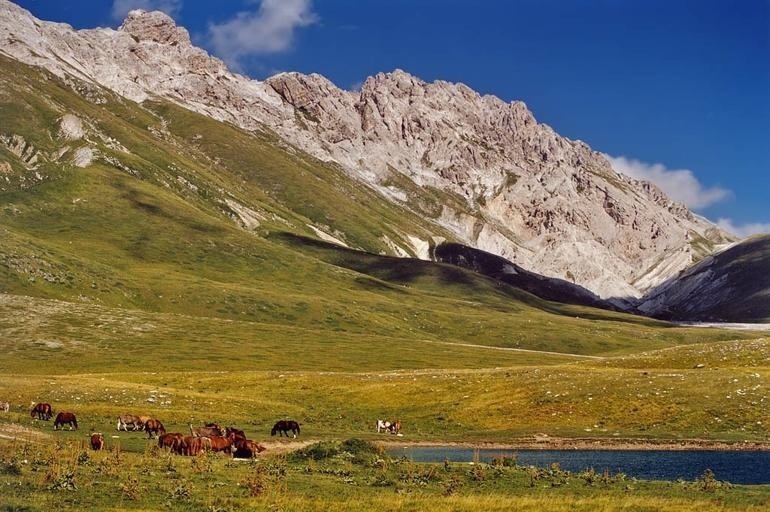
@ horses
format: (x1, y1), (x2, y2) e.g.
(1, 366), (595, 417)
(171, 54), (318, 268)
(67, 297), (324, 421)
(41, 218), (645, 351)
(4, 401), (9, 412)
(145, 419), (166, 440)
(157, 422), (266, 459)
(30, 402), (53, 421)
(117, 414), (146, 432)
(136, 415), (155, 431)
(376, 419), (393, 433)
(53, 412), (78, 431)
(392, 420), (402, 435)
(90, 432), (106, 452)
(271, 420), (300, 439)
